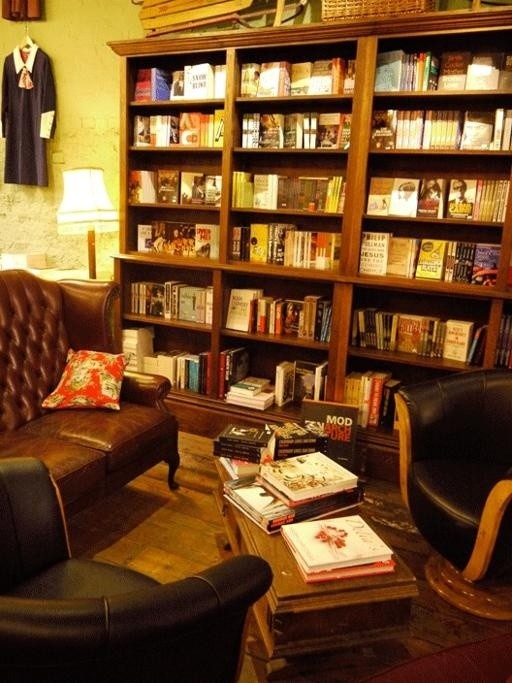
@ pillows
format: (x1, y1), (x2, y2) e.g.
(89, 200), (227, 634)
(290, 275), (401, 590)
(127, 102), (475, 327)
(41, 347), (132, 410)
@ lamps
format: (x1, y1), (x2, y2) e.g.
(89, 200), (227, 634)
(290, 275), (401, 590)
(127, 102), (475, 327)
(56, 164), (119, 280)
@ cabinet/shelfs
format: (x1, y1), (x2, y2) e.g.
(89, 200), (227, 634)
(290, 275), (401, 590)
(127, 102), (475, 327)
(105, 5), (512, 483)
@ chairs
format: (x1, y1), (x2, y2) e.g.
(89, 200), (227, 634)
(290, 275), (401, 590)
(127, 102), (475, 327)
(0, 458), (274, 681)
(392, 364), (512, 622)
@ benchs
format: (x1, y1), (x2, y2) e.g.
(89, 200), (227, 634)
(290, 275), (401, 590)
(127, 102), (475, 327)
(0, 268), (180, 494)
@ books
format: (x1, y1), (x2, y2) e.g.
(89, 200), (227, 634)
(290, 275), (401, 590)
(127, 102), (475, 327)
(211, 398), (398, 585)
(346, 369), (402, 431)
(225, 60), (356, 412)
(122, 64), (226, 395)
(357, 46), (511, 368)
